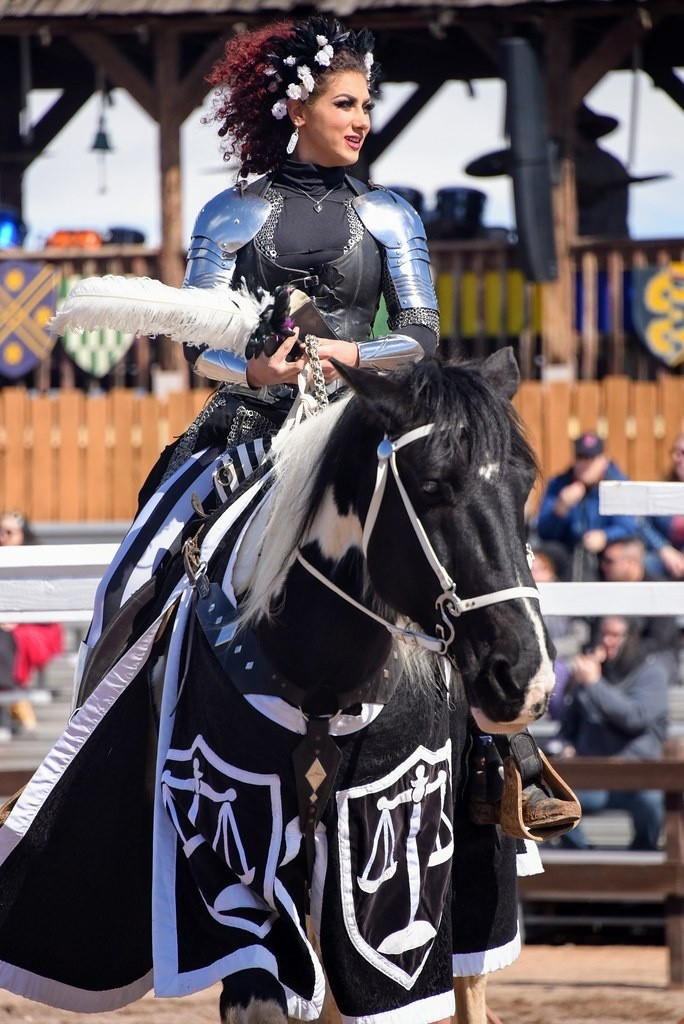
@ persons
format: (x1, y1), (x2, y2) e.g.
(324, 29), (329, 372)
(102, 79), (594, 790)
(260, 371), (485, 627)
(72, 14), (582, 843)
(529, 431), (684, 851)
(0, 506), (63, 742)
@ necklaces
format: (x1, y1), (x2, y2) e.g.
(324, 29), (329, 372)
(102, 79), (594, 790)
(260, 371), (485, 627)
(293, 182), (342, 213)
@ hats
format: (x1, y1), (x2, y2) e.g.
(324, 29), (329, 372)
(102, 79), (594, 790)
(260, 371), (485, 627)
(575, 435), (604, 457)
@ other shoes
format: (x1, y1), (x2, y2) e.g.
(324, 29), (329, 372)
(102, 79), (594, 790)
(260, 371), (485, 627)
(470, 768), (581, 830)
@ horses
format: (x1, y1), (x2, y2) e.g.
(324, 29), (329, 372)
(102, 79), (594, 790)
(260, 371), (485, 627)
(110, 345), (560, 1024)
